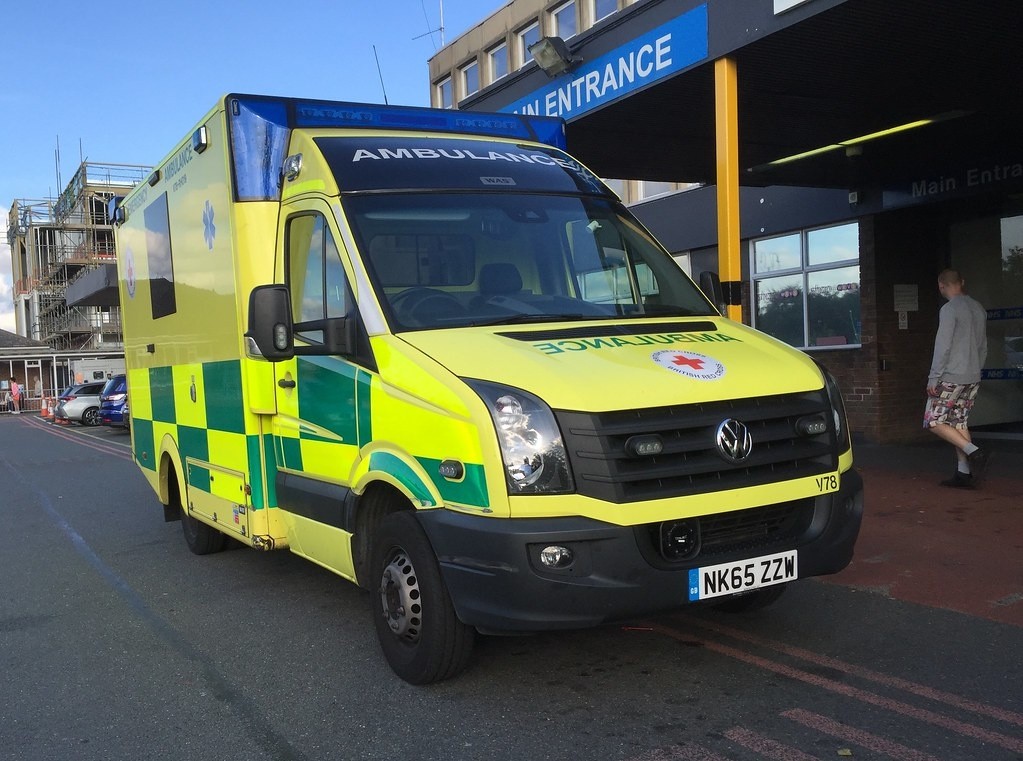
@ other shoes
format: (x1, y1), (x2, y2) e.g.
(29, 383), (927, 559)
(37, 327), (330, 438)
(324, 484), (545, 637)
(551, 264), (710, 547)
(11, 411), (21, 415)
(940, 470), (972, 487)
(968, 447), (987, 488)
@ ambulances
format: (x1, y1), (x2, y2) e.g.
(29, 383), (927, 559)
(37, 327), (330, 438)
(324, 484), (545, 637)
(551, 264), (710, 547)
(110, 87), (864, 688)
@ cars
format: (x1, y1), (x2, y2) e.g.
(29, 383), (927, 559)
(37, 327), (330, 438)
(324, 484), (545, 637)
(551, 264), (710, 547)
(54, 381), (107, 427)
(122, 399), (130, 431)
(98, 373), (127, 427)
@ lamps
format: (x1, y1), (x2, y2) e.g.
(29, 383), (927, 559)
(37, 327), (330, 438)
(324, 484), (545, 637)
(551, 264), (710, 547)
(527, 37), (584, 79)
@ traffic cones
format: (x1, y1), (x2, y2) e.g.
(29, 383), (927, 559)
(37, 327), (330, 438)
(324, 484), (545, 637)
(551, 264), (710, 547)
(38, 393), (48, 417)
(55, 419), (72, 425)
(47, 396), (55, 419)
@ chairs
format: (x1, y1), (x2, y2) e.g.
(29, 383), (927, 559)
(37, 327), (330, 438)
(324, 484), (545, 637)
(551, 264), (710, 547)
(470, 263), (541, 311)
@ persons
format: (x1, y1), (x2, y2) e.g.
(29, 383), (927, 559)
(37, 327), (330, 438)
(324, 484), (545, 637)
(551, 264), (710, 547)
(10, 377), (20, 414)
(34, 376), (41, 398)
(922, 269), (994, 490)
